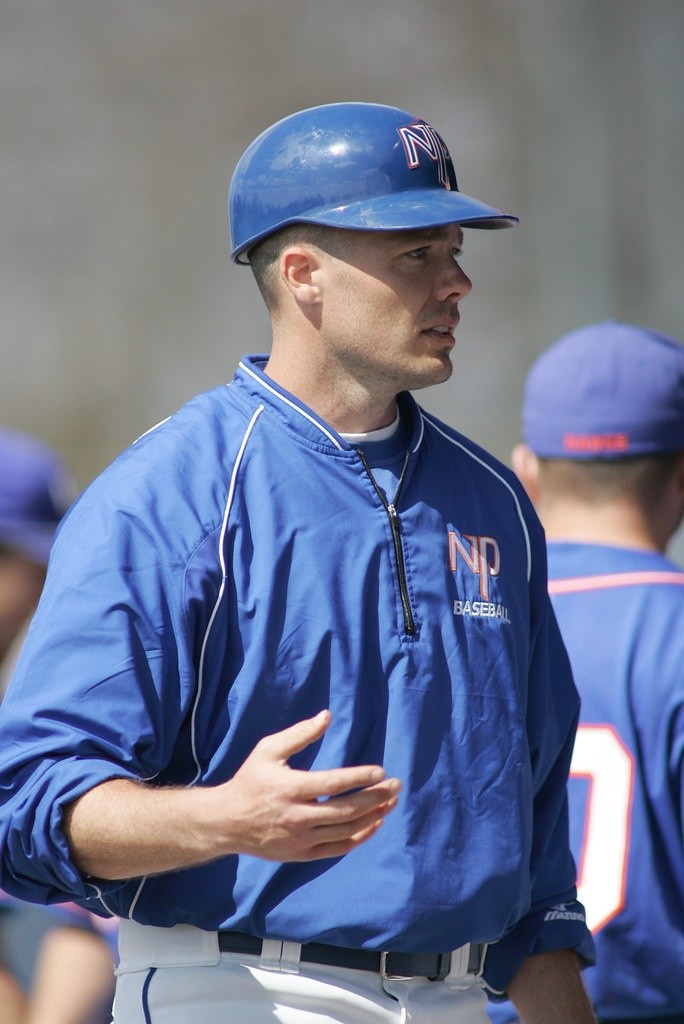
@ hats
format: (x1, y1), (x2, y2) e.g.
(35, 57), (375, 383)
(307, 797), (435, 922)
(0, 424), (77, 566)
(522, 320), (684, 458)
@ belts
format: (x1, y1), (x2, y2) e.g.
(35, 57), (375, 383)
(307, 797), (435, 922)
(218, 930), (482, 978)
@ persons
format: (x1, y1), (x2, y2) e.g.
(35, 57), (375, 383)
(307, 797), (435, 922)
(0, 426), (121, 1024)
(483, 317), (684, 1024)
(0, 98), (604, 1024)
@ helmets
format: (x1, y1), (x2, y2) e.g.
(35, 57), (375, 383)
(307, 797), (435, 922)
(229, 101), (519, 266)
